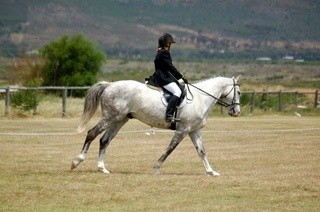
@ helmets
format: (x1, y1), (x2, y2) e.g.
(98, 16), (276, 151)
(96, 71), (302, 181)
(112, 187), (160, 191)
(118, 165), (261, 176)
(158, 33), (176, 50)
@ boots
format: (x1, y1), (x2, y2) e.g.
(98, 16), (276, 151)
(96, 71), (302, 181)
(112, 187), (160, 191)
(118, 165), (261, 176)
(166, 95), (181, 122)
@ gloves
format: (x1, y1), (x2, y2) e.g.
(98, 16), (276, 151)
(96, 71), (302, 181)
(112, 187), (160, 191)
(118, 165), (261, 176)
(182, 78), (188, 84)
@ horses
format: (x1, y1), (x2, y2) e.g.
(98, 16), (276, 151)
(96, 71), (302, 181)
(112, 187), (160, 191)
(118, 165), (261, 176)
(71, 77), (240, 177)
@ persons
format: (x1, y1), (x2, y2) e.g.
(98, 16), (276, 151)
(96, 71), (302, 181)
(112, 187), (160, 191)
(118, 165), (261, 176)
(145, 33), (188, 123)
